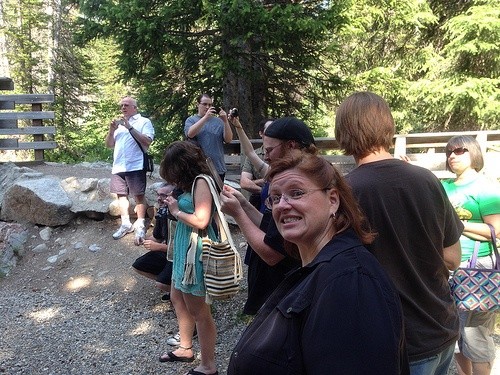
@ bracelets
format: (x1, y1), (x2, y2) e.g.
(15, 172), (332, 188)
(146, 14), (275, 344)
(176, 211), (182, 219)
(129, 126), (133, 131)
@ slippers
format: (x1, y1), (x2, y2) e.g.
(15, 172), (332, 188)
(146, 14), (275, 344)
(159, 352), (194, 362)
(183, 368), (219, 375)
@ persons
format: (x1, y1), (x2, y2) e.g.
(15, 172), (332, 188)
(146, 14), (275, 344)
(132, 139), (219, 375)
(219, 118), (317, 316)
(334, 91), (465, 375)
(440, 134), (500, 375)
(228, 107), (271, 179)
(225, 148), (406, 375)
(105, 97), (155, 239)
(240, 119), (276, 317)
(184, 94), (233, 183)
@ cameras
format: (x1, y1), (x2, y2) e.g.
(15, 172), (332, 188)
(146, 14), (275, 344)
(231, 109), (238, 117)
(212, 105), (220, 113)
(116, 119), (123, 124)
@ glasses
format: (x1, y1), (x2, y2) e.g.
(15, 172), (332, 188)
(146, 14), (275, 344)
(200, 102), (214, 108)
(265, 187), (332, 209)
(445, 147), (466, 158)
(264, 141), (288, 157)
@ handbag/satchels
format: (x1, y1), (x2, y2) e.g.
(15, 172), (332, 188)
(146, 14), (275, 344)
(190, 174), (243, 304)
(448, 222), (499, 312)
(144, 153), (154, 172)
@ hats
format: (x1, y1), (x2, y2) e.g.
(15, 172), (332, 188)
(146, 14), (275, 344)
(263, 117), (316, 149)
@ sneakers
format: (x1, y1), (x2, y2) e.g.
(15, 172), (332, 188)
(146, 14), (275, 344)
(167, 331), (182, 345)
(112, 223), (133, 240)
(133, 218), (147, 239)
(161, 293), (172, 303)
